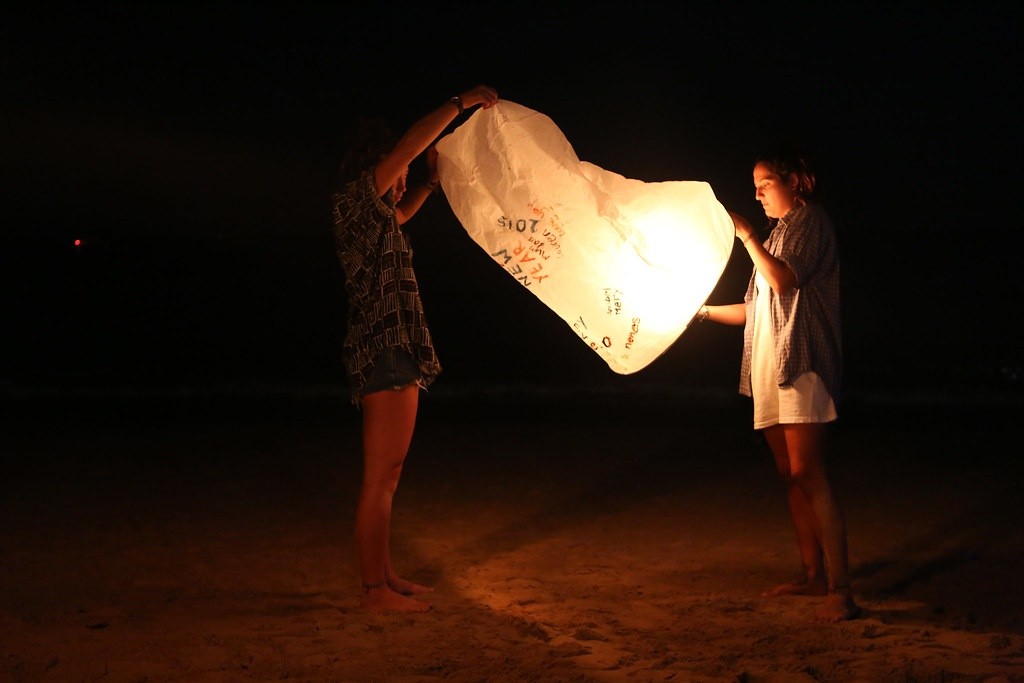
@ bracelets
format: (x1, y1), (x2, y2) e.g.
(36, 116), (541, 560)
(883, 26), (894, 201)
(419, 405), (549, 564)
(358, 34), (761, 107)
(699, 312), (709, 323)
(422, 178), (437, 189)
(744, 232), (758, 243)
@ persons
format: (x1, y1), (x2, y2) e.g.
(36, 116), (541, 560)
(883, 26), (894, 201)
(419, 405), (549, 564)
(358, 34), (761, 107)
(694, 150), (859, 624)
(328, 85), (498, 614)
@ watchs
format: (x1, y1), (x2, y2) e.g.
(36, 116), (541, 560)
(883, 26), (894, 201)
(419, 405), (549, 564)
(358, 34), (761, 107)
(450, 95), (464, 115)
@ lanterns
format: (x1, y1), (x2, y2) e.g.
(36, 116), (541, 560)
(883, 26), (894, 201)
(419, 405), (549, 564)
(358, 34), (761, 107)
(435, 98), (735, 375)
